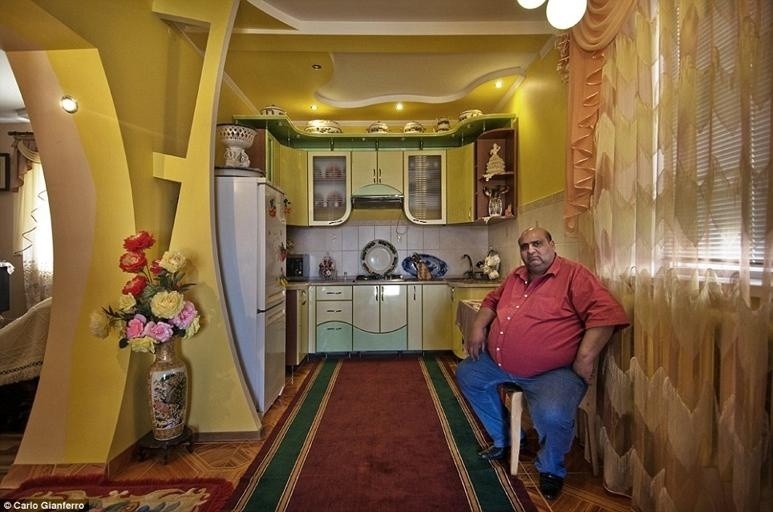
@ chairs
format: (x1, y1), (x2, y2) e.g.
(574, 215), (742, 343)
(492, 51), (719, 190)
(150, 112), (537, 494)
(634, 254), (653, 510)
(0, 298), (52, 435)
(500, 354), (600, 479)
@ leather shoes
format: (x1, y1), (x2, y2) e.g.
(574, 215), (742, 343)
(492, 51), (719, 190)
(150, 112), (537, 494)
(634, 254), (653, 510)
(477, 441), (527, 461)
(540, 473), (563, 500)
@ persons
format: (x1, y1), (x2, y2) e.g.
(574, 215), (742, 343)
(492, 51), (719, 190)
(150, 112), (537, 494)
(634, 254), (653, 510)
(485, 142), (505, 174)
(453, 227), (631, 500)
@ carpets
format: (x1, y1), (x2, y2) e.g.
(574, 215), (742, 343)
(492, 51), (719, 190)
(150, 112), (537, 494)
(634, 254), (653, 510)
(220, 353), (538, 512)
(0, 475), (233, 512)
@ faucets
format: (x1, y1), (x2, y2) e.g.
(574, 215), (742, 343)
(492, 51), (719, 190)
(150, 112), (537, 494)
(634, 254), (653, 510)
(462, 253), (473, 278)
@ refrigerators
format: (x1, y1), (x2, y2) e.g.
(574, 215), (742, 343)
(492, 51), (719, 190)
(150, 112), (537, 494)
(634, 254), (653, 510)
(214, 176), (286, 417)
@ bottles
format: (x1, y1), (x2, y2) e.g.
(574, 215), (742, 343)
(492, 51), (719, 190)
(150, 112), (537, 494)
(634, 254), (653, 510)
(343, 272), (348, 282)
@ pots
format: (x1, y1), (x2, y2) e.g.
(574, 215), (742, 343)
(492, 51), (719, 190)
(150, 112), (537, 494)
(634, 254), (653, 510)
(366, 121), (392, 134)
(400, 121), (427, 133)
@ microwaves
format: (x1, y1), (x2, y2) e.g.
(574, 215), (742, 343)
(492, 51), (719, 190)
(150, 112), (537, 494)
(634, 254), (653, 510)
(283, 253), (310, 281)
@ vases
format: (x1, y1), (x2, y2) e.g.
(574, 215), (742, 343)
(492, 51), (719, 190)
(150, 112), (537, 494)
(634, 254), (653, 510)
(144, 337), (188, 441)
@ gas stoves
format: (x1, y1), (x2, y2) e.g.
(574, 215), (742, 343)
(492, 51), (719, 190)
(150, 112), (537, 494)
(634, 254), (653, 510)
(353, 274), (406, 282)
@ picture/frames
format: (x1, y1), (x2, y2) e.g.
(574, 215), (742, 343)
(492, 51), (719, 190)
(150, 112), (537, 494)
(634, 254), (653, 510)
(0, 153), (10, 191)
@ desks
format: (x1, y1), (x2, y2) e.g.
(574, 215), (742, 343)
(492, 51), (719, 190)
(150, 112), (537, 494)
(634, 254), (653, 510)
(456, 299), (485, 360)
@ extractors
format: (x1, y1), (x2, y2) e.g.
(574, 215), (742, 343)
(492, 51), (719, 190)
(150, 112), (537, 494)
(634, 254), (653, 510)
(353, 197), (401, 209)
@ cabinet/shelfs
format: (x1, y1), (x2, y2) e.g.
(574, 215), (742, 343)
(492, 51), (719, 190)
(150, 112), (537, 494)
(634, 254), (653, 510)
(449, 285), (492, 360)
(475, 127), (518, 224)
(307, 151), (351, 227)
(236, 127), (280, 181)
(403, 148), (445, 226)
(279, 143), (308, 227)
(308, 286), (352, 353)
(445, 143), (475, 224)
(351, 148), (403, 197)
(351, 285), (407, 351)
(286, 289), (309, 366)
(407, 284), (453, 351)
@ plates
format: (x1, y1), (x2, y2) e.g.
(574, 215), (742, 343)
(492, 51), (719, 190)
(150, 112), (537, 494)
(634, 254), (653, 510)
(360, 240), (399, 276)
(402, 254), (448, 277)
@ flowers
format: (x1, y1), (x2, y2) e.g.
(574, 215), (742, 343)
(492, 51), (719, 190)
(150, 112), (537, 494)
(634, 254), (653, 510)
(87, 230), (200, 352)
